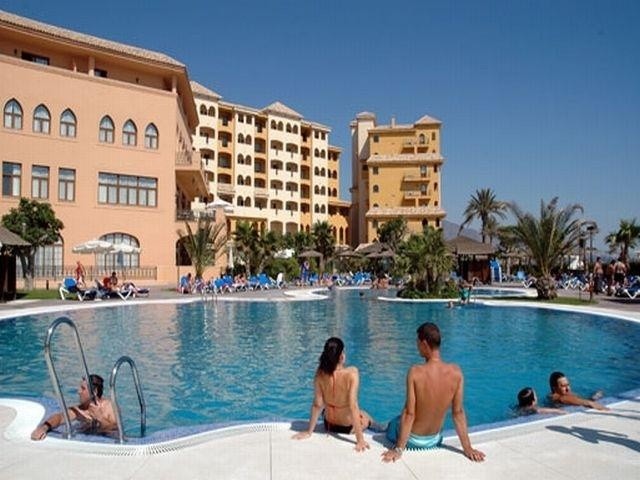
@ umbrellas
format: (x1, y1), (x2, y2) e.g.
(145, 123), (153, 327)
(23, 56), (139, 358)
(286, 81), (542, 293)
(71, 239), (112, 278)
(109, 242), (142, 277)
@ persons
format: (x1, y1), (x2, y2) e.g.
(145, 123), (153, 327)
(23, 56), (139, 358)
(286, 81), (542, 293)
(291, 338), (381, 453)
(105, 271), (119, 291)
(455, 280), (473, 304)
(121, 281), (140, 295)
(592, 256), (604, 294)
(30, 373), (119, 441)
(613, 256), (627, 293)
(381, 320), (486, 465)
(520, 270), (591, 291)
(178, 271), (249, 295)
(275, 270), (392, 291)
(603, 259), (615, 297)
(512, 386), (569, 416)
(545, 370), (611, 411)
(73, 261), (87, 287)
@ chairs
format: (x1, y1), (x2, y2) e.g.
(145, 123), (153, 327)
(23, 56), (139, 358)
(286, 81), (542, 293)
(58, 275), (150, 302)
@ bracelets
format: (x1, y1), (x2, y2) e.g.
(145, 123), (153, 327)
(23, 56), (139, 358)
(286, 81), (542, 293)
(394, 447), (402, 455)
(44, 421), (52, 432)
